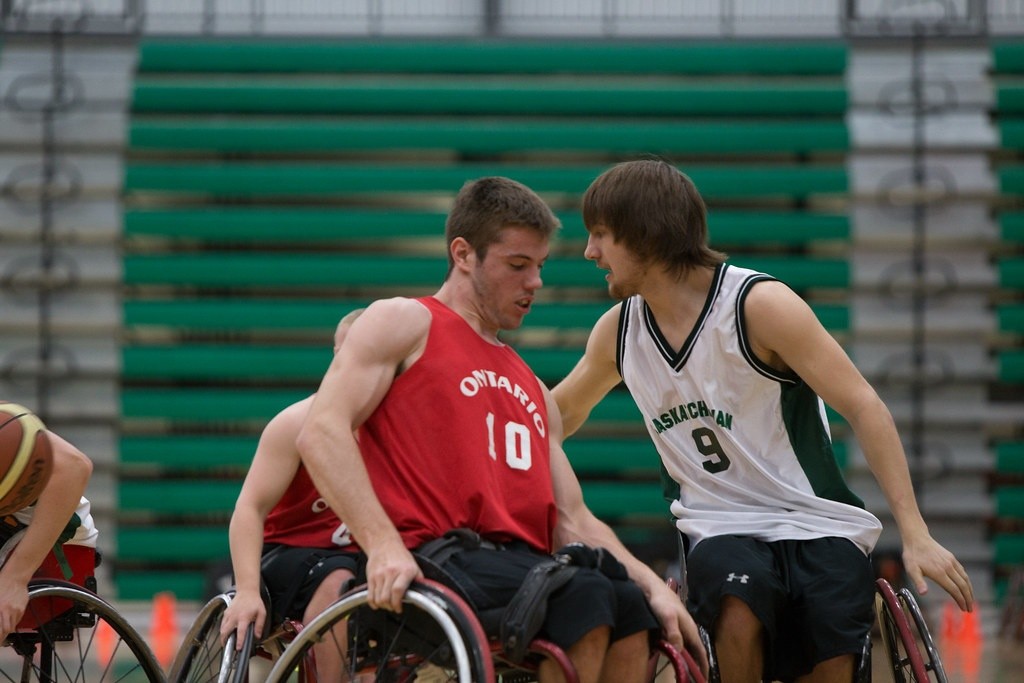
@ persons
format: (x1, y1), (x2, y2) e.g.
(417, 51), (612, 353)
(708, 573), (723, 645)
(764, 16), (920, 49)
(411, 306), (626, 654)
(0, 178), (710, 683)
(538, 162), (979, 683)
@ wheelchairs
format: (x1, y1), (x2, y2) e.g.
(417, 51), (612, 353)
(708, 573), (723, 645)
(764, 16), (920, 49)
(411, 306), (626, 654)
(167, 519), (947, 683)
(1, 575), (168, 683)
